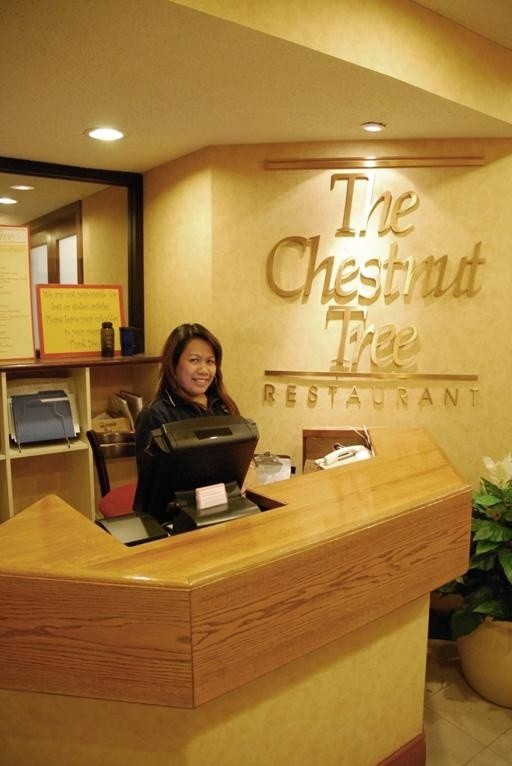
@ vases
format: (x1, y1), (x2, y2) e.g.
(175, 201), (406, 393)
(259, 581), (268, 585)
(450, 607), (511, 712)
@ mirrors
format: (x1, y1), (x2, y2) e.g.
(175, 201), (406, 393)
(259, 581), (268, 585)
(0, 158), (143, 360)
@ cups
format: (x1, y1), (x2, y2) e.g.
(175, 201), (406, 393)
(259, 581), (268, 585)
(118, 326), (136, 356)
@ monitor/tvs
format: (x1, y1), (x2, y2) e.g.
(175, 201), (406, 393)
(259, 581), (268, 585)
(131, 415), (261, 531)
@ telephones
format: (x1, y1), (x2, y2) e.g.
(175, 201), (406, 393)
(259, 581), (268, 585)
(320, 445), (371, 469)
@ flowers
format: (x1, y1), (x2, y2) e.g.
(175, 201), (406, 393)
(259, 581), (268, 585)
(428, 452), (511, 636)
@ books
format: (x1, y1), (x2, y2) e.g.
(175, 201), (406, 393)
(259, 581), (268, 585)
(112, 390), (143, 432)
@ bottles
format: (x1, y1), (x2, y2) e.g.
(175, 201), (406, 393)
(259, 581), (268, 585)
(100, 321), (115, 357)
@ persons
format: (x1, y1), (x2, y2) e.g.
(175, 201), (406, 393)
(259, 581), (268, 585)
(131, 322), (241, 524)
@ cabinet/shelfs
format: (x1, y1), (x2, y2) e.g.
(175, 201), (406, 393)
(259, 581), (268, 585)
(1, 353), (164, 533)
